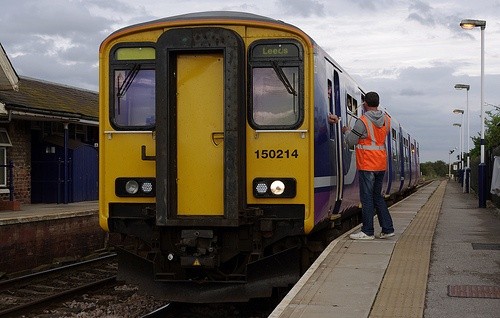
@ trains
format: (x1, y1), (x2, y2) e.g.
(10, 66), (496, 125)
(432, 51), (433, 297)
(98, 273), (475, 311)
(98, 11), (420, 301)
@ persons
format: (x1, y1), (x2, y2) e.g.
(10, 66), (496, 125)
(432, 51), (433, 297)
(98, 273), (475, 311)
(328, 85), (339, 124)
(343, 92), (396, 240)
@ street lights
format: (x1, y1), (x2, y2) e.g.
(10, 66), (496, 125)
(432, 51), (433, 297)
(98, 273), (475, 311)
(459, 19), (487, 208)
(454, 84), (470, 193)
(452, 123), (462, 186)
(453, 109), (464, 188)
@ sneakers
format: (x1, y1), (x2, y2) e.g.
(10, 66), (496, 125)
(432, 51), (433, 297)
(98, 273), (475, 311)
(350, 231), (375, 240)
(376, 232), (396, 239)
